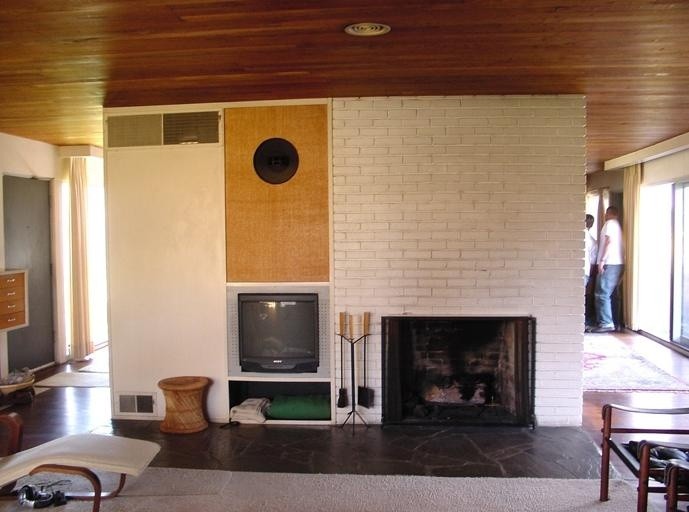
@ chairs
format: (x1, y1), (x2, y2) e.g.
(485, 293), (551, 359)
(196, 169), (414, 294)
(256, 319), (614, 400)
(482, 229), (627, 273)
(602, 401), (689, 511)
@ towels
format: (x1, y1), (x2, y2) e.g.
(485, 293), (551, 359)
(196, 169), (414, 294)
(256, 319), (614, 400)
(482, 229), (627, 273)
(229, 397), (269, 425)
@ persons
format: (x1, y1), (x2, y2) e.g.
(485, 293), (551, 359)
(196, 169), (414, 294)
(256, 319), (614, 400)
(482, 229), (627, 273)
(585, 214), (599, 319)
(590, 204), (626, 334)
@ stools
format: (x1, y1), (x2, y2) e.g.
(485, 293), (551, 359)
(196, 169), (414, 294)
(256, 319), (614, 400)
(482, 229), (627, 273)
(157, 376), (213, 435)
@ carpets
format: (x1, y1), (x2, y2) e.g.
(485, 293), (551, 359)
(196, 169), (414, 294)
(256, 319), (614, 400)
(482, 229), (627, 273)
(34, 370), (112, 389)
(583, 331), (688, 391)
(1, 462), (668, 511)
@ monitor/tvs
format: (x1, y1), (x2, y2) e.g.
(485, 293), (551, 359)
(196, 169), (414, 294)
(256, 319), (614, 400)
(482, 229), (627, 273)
(238, 293), (319, 373)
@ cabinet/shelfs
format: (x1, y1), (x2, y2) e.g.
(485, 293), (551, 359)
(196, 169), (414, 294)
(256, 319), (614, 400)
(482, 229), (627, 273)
(0, 267), (31, 335)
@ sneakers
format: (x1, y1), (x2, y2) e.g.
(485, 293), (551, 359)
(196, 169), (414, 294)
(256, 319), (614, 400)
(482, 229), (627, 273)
(0, 386), (36, 405)
(591, 325), (615, 333)
